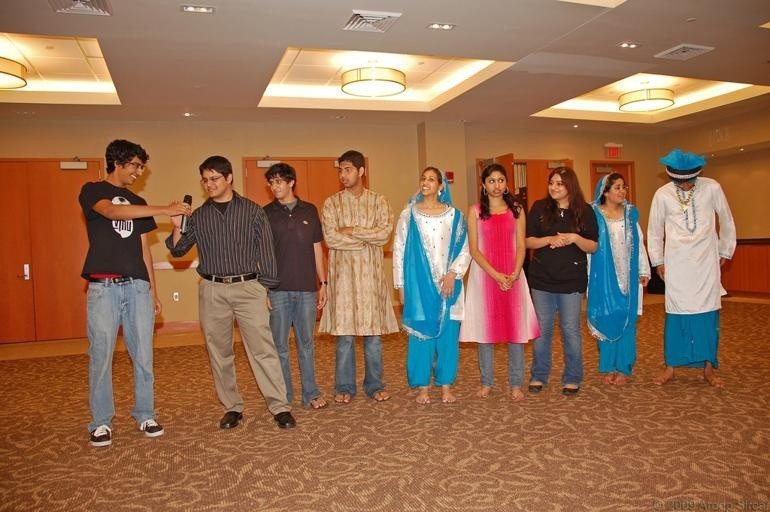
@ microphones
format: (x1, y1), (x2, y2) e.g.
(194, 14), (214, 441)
(179, 193), (193, 235)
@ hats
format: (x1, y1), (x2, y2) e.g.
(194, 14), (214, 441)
(659, 148), (706, 182)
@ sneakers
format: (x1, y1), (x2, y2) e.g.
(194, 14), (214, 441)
(90, 425), (112, 447)
(138, 419), (164, 437)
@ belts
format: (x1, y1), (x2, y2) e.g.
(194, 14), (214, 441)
(87, 276), (143, 284)
(199, 272), (257, 284)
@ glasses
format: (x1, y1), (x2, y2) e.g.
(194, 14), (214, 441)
(267, 179), (284, 186)
(116, 161), (147, 172)
(336, 167), (352, 174)
(200, 175), (224, 185)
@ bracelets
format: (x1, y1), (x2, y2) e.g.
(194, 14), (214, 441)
(319, 281), (327, 286)
(511, 272), (520, 281)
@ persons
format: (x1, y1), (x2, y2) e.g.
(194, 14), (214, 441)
(391, 165), (473, 405)
(646, 148), (737, 389)
(317, 150), (400, 404)
(262, 162), (329, 409)
(585, 171), (652, 388)
(164, 155), (297, 430)
(523, 166), (599, 395)
(78, 138), (194, 447)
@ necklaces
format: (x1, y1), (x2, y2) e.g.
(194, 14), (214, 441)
(676, 184), (696, 209)
(599, 206), (625, 221)
(685, 197), (697, 233)
(457, 164), (541, 403)
(559, 208), (565, 218)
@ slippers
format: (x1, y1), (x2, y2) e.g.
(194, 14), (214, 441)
(334, 393), (352, 405)
(374, 391), (392, 401)
(311, 397), (328, 410)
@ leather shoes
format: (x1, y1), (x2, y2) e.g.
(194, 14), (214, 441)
(274, 411), (296, 428)
(528, 384), (543, 392)
(563, 386), (580, 394)
(220, 411), (243, 429)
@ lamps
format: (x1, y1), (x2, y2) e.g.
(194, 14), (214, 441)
(0, 57), (28, 91)
(618, 84), (675, 113)
(341, 68), (406, 98)
